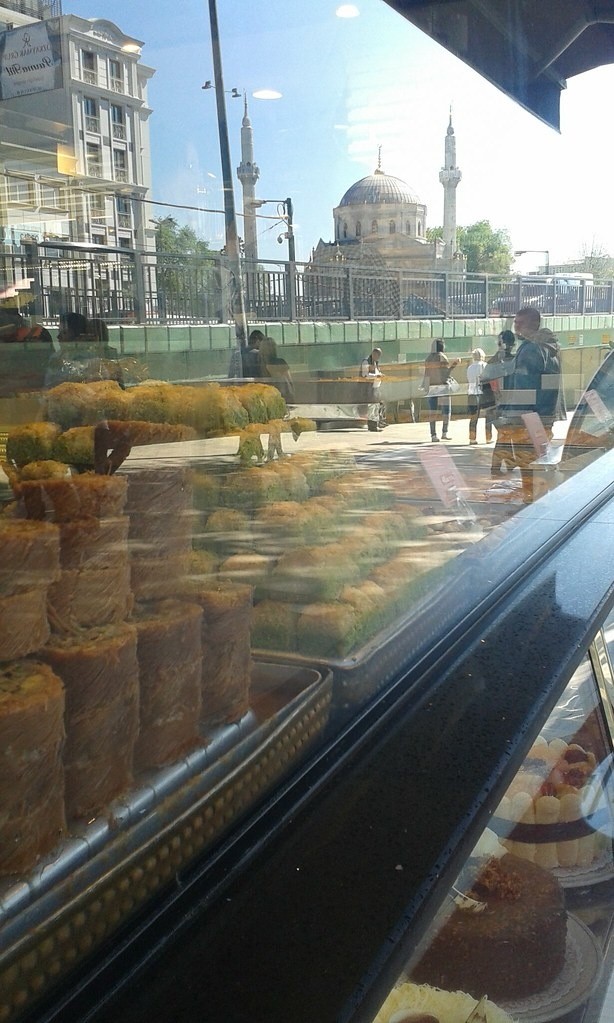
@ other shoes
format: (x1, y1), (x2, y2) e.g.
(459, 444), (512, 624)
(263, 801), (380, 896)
(369, 426), (383, 432)
(487, 441), (495, 444)
(506, 462), (517, 471)
(470, 441), (478, 445)
(491, 470), (505, 476)
(441, 432), (452, 440)
(432, 437), (440, 442)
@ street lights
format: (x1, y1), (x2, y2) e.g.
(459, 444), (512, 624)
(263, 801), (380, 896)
(514, 250), (549, 313)
(202, 0), (250, 357)
(246, 197), (298, 321)
(148, 214), (173, 324)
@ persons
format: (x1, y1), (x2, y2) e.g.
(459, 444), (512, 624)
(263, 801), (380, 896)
(466, 348), (495, 446)
(418, 338), (461, 442)
(225, 329), (266, 467)
(358, 347), (389, 432)
(0, 305), (56, 469)
(255, 337), (296, 464)
(33, 313), (109, 473)
(484, 330), (520, 476)
(87, 318), (133, 477)
(500, 307), (569, 507)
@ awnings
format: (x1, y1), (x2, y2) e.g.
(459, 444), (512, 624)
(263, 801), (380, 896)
(383, 0), (614, 135)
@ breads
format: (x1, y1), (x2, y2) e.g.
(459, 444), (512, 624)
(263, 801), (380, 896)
(0, 382), (614, 903)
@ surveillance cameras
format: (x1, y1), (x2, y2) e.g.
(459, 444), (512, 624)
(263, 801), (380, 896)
(277, 237), (283, 244)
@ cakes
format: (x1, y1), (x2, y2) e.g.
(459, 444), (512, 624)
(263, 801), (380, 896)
(372, 666), (614, 1023)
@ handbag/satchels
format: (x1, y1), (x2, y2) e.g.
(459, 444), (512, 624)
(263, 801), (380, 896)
(480, 382), (496, 410)
(422, 357), (430, 397)
(444, 374), (461, 393)
